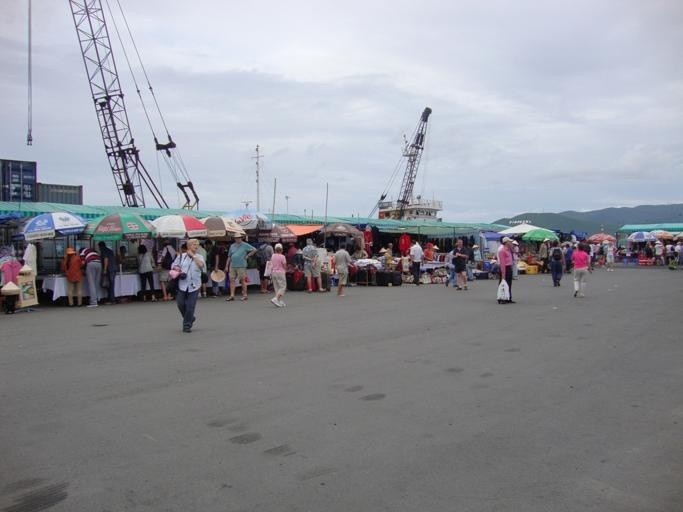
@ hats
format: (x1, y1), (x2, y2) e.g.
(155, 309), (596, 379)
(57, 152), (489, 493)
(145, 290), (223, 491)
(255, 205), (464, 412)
(211, 269), (225, 282)
(654, 240), (660, 244)
(543, 238), (550, 242)
(66, 248), (76, 254)
(232, 232), (245, 238)
(502, 236), (512, 243)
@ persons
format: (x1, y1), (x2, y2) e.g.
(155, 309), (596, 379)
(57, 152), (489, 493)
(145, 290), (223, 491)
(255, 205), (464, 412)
(137, 239), (187, 302)
(287, 239), (325, 292)
(61, 241), (128, 307)
(200, 233), (257, 301)
(632, 243), (682, 268)
(257, 240), (293, 307)
(333, 244), (352, 296)
(445, 240), (481, 291)
(380, 243), (394, 269)
(410, 239), (438, 286)
(538, 238), (617, 297)
(496, 237), (518, 305)
(351, 246), (368, 260)
(172, 238), (204, 332)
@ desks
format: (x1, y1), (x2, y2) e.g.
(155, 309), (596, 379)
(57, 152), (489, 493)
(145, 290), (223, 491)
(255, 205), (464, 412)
(42, 268), (260, 304)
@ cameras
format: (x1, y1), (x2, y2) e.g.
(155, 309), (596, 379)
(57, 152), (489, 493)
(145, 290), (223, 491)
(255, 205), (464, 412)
(177, 272), (187, 280)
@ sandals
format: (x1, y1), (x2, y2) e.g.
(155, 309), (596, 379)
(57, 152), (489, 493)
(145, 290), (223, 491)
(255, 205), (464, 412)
(226, 296), (234, 300)
(240, 296), (248, 301)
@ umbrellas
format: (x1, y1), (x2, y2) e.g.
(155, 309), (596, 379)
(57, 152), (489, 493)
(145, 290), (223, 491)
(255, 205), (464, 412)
(628, 227), (683, 246)
(21, 209), (273, 267)
(589, 230), (616, 244)
(521, 228), (561, 244)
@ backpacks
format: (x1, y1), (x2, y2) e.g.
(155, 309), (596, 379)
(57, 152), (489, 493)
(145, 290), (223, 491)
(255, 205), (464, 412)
(553, 248), (562, 260)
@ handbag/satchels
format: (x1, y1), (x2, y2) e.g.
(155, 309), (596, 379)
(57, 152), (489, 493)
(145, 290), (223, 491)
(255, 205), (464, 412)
(101, 275), (110, 288)
(201, 271), (208, 283)
(163, 248), (174, 270)
(264, 260), (272, 277)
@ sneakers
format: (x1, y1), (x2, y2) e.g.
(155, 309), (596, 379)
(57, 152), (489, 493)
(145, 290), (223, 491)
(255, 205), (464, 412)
(270, 298), (285, 307)
